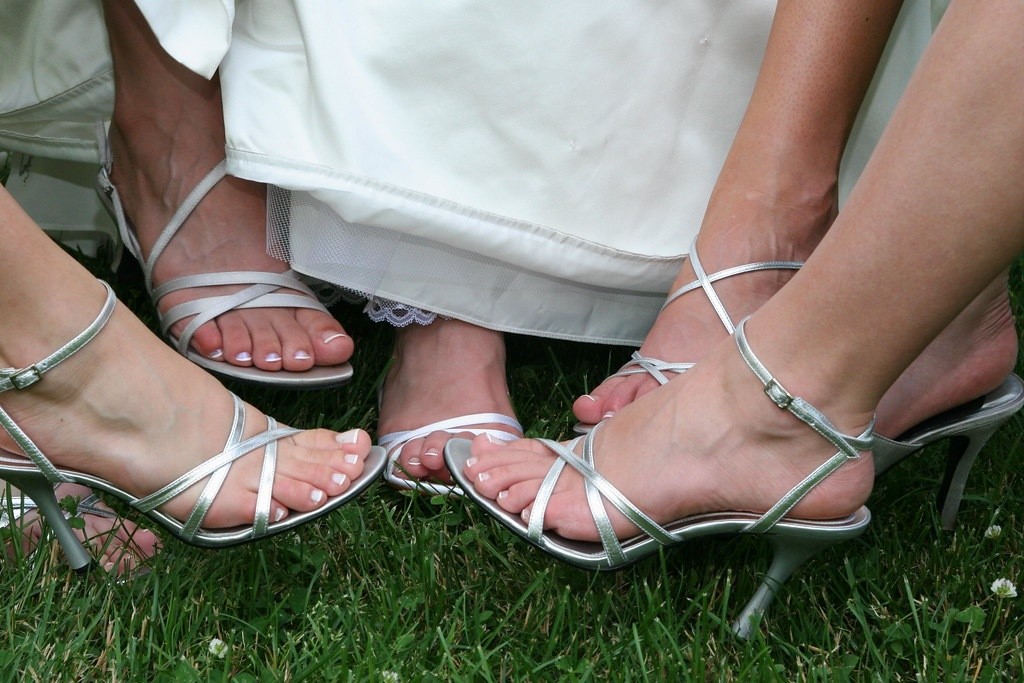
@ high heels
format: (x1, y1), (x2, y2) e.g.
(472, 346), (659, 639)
(95, 114), (356, 383)
(873, 372), (1024, 533)
(442, 315), (872, 640)
(0, 277), (388, 569)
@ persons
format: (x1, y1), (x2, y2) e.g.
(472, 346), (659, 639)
(0, 0), (1024, 643)
(443, 0), (1024, 642)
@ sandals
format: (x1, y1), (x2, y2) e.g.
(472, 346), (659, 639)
(375, 354), (522, 499)
(2, 482), (164, 586)
(571, 235), (804, 441)
